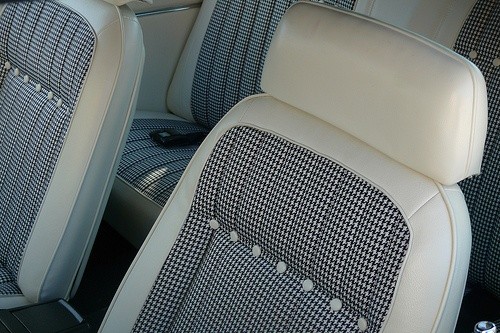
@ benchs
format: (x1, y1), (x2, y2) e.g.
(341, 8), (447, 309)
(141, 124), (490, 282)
(103, 0), (375, 252)
(98, 2), (487, 332)
(0, 0), (146, 312)
(432, 0), (498, 301)
